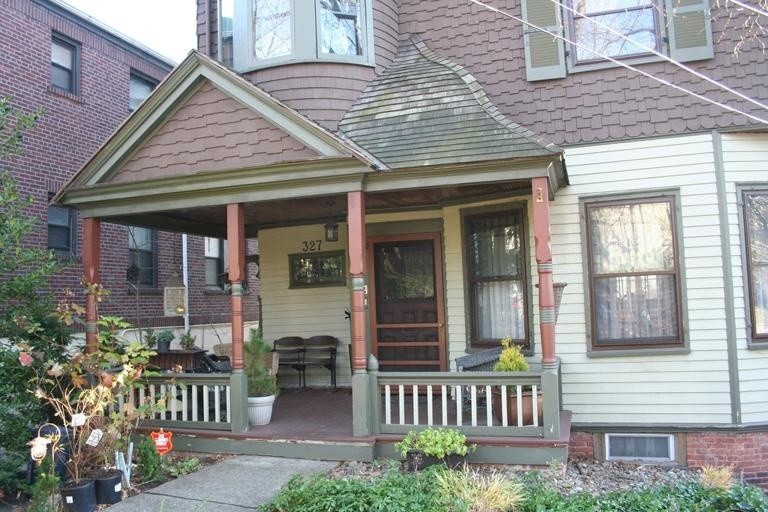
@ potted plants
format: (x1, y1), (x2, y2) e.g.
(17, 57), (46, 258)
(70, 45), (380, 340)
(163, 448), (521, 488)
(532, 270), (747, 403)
(243, 328), (280, 426)
(394, 426), (477, 473)
(491, 338), (543, 427)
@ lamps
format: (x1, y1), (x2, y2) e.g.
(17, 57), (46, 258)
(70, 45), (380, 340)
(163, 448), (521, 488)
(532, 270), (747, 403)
(324, 200), (339, 242)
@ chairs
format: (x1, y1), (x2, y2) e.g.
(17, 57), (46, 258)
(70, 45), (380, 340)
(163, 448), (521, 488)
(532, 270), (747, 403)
(214, 342), (279, 389)
(274, 336), (339, 394)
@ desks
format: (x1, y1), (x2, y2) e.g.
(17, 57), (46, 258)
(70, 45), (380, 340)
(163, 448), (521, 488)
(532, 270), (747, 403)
(134, 349), (208, 408)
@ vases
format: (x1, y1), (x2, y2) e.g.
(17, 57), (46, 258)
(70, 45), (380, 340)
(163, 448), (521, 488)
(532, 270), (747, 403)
(10, 282), (186, 512)
